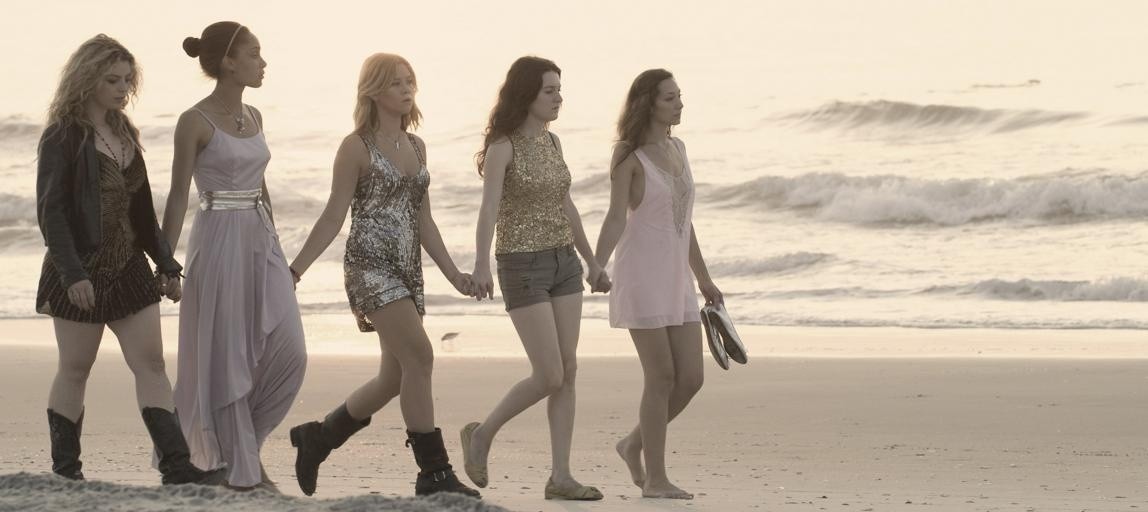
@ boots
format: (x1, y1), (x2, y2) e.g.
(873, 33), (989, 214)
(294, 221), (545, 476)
(44, 409), (87, 480)
(404, 430), (479, 500)
(137, 409), (230, 487)
(290, 406), (369, 497)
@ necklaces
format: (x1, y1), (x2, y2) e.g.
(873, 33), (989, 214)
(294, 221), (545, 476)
(95, 126), (125, 171)
(212, 91), (247, 131)
(379, 127), (402, 149)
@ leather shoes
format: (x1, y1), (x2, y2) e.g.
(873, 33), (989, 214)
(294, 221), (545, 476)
(463, 422), (494, 490)
(546, 477), (601, 503)
(711, 304), (750, 368)
(701, 308), (732, 374)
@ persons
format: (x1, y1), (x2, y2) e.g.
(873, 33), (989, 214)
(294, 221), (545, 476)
(34, 34), (221, 485)
(588, 71), (722, 496)
(290, 54), (481, 496)
(463, 58), (601, 505)
(157, 20), (305, 490)
